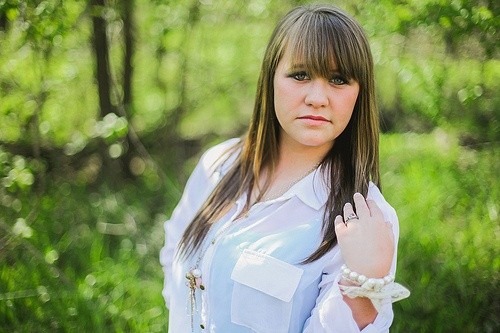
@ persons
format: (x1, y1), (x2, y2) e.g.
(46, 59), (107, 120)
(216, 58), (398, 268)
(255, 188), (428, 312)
(158, 7), (412, 333)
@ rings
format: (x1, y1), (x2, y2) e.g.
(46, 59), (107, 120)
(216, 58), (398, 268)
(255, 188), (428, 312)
(344, 214), (358, 224)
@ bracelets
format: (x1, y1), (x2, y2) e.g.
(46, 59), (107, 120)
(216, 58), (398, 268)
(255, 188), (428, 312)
(337, 266), (411, 314)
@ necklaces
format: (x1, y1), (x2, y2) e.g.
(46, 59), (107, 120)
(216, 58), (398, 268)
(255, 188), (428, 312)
(235, 161), (322, 219)
